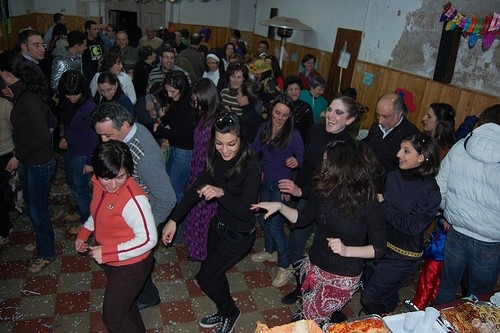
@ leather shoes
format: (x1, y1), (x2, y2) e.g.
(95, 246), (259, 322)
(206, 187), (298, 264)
(138, 294), (159, 309)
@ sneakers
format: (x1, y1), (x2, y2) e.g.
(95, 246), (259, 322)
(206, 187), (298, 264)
(404, 299), (418, 310)
(63, 210), (83, 222)
(24, 240), (37, 251)
(68, 224), (86, 236)
(272, 264), (294, 287)
(29, 256), (55, 273)
(198, 307), (241, 333)
(251, 247), (278, 262)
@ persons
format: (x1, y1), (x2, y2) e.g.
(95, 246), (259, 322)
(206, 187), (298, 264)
(0, 13), (500, 333)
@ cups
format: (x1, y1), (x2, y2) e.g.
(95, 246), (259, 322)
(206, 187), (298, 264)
(403, 312), (418, 332)
(424, 307), (439, 328)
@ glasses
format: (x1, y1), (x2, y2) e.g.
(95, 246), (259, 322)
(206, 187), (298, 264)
(30, 41), (48, 48)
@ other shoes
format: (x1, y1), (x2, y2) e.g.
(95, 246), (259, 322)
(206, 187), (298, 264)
(282, 286), (305, 304)
(293, 307), (305, 321)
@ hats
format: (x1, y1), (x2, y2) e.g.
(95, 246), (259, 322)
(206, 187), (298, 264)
(247, 58), (273, 73)
